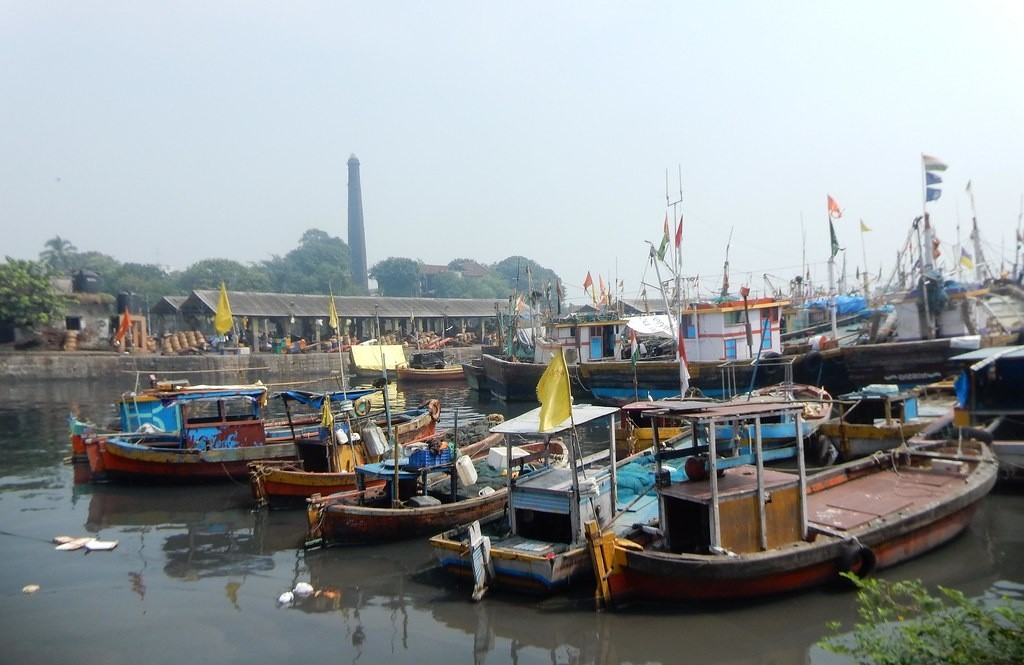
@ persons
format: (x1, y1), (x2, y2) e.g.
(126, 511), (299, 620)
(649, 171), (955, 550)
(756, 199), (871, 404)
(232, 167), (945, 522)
(239, 329), (243, 340)
(262, 330), (276, 340)
(228, 330), (232, 340)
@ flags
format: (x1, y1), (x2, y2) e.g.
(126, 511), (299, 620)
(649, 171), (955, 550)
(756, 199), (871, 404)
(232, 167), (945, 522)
(116, 305), (133, 340)
(515, 291), (525, 313)
(322, 399), (334, 427)
(630, 328), (640, 370)
(827, 194), (841, 259)
(328, 293), (339, 328)
(679, 322), (690, 400)
(675, 215), (684, 248)
(536, 346), (571, 432)
(924, 154), (947, 202)
(657, 217), (671, 260)
(860, 222), (871, 232)
(583, 271), (624, 306)
(961, 255), (973, 268)
(410, 310), (415, 322)
(542, 278), (564, 302)
(215, 280), (233, 335)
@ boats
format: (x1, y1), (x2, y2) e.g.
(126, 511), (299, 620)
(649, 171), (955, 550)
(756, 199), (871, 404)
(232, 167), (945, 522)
(606, 400), (717, 454)
(392, 277), (1024, 485)
(304, 433), (569, 542)
(241, 398), (442, 507)
(603, 391), (998, 606)
(68, 378), (340, 483)
(425, 370), (829, 584)
(818, 370), (968, 464)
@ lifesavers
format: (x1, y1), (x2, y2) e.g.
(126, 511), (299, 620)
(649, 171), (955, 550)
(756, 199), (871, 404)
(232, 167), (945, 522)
(354, 396), (372, 417)
(417, 395), (443, 422)
